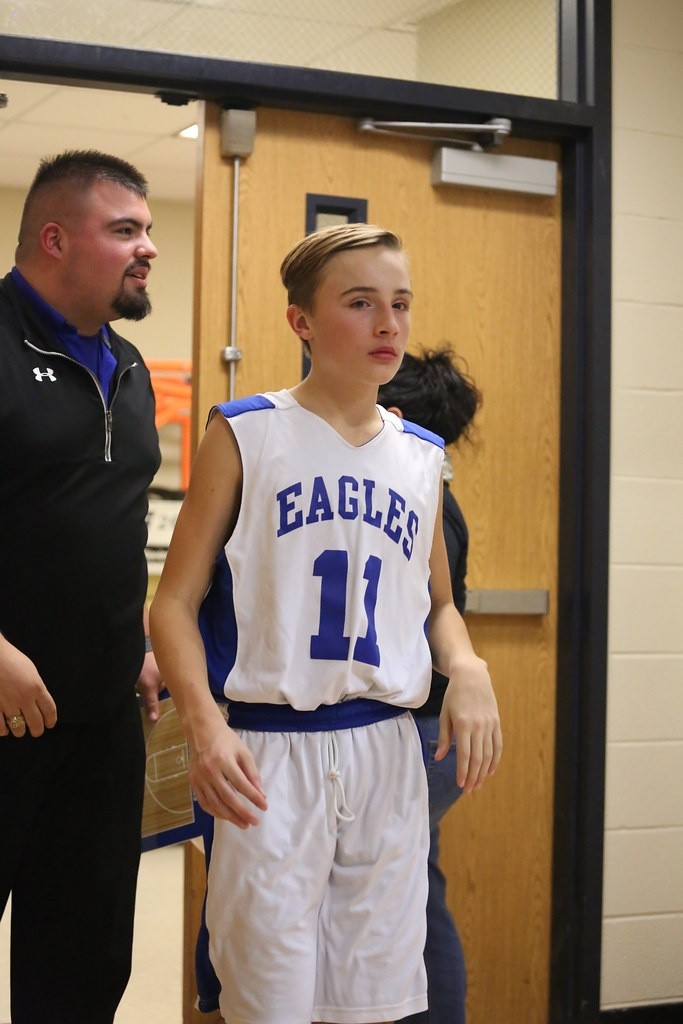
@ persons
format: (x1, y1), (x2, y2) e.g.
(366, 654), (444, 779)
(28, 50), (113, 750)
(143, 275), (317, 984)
(0, 150), (163, 1024)
(149, 222), (501, 1024)
(196, 350), (477, 1024)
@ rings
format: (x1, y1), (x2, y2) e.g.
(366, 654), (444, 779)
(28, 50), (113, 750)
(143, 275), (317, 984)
(7, 716), (23, 728)
